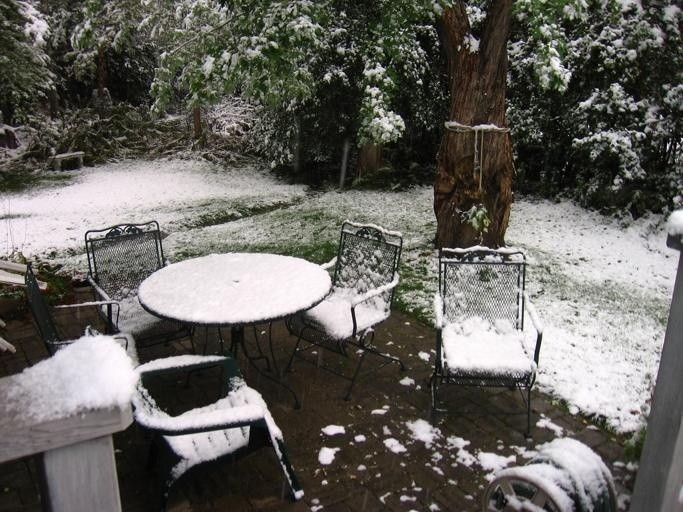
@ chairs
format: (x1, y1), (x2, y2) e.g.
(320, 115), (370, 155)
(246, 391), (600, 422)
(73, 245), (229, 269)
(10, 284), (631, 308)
(427, 245), (543, 440)
(85, 220), (197, 356)
(131, 356), (305, 503)
(22, 261), (128, 352)
(287, 219), (408, 401)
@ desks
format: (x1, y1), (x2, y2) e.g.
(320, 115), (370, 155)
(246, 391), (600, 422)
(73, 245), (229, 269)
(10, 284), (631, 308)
(138, 252), (333, 410)
